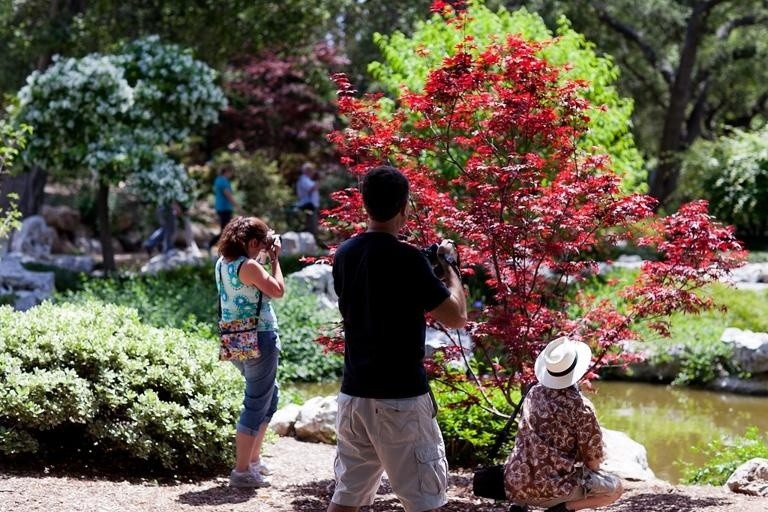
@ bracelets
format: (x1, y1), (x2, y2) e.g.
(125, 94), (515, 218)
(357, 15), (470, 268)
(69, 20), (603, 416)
(268, 259), (279, 264)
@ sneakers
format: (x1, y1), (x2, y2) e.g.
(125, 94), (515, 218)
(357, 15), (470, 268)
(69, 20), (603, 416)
(229, 458), (275, 488)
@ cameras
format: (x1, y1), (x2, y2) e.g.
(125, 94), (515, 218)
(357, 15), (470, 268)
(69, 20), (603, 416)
(421, 238), (458, 278)
(266, 233), (282, 250)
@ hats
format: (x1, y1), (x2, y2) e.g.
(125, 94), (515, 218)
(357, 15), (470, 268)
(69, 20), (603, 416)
(533, 336), (593, 390)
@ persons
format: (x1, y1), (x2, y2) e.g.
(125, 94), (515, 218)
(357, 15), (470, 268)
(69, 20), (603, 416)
(210, 214), (288, 489)
(168, 183), (197, 251)
(499, 336), (626, 511)
(324, 163), (470, 512)
(203, 162), (247, 251)
(141, 150), (183, 259)
(294, 160), (331, 251)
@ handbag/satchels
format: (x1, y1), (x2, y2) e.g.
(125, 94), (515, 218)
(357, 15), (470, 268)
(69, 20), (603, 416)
(473, 463), (507, 501)
(218, 317), (262, 363)
